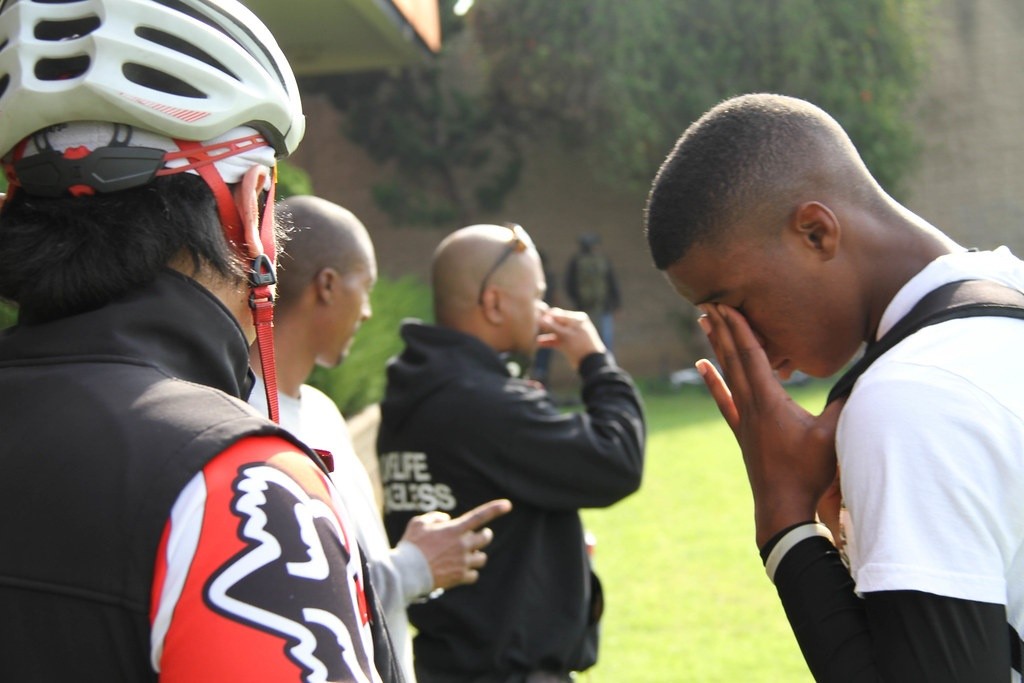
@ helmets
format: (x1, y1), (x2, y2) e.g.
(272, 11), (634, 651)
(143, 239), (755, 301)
(0, 0), (306, 191)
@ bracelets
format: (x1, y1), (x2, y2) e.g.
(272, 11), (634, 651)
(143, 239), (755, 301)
(765, 524), (839, 595)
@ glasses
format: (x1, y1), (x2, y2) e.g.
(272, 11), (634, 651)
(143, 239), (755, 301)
(478, 222), (532, 305)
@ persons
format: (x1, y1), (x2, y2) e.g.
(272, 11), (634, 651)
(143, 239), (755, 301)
(0, 0), (404, 683)
(647, 94), (1024, 683)
(245, 193), (512, 683)
(534, 251), (558, 381)
(566, 232), (621, 351)
(377, 223), (647, 683)
